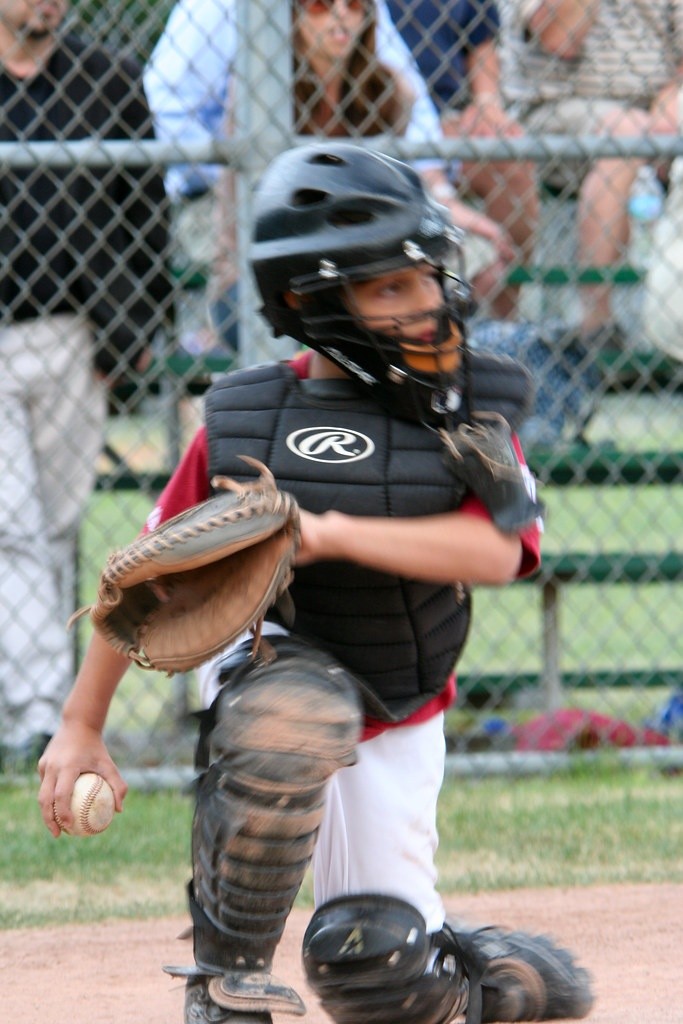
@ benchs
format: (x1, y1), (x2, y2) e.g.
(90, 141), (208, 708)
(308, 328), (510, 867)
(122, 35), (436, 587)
(87, 174), (679, 686)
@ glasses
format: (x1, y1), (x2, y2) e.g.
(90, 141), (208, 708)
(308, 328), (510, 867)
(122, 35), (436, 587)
(300, 0), (370, 13)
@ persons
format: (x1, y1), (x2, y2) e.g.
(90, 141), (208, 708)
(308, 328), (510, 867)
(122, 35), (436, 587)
(183, 0), (413, 372)
(143, 0), (524, 389)
(384, 1), (541, 319)
(499, 1), (683, 385)
(0, 1), (169, 775)
(38, 140), (592, 1024)
(645, 163), (682, 385)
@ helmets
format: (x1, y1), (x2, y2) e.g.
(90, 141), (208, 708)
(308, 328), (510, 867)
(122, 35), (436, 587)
(248, 139), (475, 434)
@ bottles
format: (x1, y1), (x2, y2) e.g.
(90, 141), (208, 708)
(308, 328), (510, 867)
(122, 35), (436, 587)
(626, 166), (666, 275)
(170, 205), (216, 355)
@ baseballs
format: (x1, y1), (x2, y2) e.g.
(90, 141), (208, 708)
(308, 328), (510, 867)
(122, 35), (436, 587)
(62, 773), (119, 841)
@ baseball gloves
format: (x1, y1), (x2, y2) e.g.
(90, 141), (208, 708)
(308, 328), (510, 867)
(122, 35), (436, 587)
(86, 488), (306, 676)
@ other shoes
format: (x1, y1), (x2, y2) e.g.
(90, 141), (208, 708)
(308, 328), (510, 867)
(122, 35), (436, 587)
(461, 918), (593, 1022)
(170, 964), (305, 1024)
